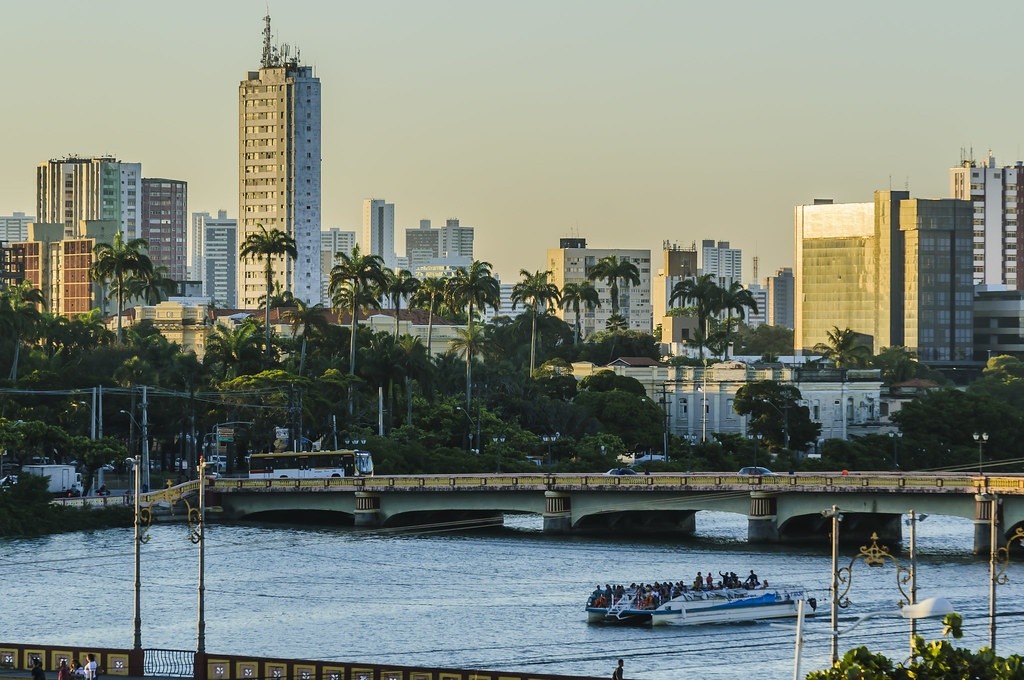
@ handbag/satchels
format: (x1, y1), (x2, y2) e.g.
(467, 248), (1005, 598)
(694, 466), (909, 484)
(95, 664), (103, 676)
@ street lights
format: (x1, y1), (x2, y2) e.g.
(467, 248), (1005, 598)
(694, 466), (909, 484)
(353, 438), (367, 476)
(119, 409), (149, 492)
(888, 430), (903, 471)
(761, 397), (790, 447)
(542, 433), (559, 476)
(456, 406), (481, 455)
(972, 431), (989, 473)
(468, 433), (473, 455)
(492, 434), (507, 476)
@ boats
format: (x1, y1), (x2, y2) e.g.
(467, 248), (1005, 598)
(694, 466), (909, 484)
(585, 582), (817, 627)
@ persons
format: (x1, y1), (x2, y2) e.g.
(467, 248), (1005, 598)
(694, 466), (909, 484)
(590, 569), (770, 609)
(612, 659), (624, 680)
(31, 659), (46, 680)
(84, 654), (97, 680)
(617, 468), (625, 475)
(66, 484), (80, 497)
(100, 483), (106, 490)
(4, 476), (12, 486)
(645, 468), (650, 475)
(58, 660), (71, 680)
(69, 659), (84, 680)
(176, 471), (189, 485)
(842, 468), (848, 475)
(789, 467), (794, 475)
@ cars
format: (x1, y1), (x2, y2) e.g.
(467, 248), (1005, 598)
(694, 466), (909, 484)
(0, 475), (19, 492)
(737, 467), (778, 475)
(603, 468), (639, 475)
(101, 464), (115, 472)
(174, 454), (250, 472)
(634, 455), (666, 464)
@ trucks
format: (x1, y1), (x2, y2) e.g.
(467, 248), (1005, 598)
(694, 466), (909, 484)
(21, 465), (85, 496)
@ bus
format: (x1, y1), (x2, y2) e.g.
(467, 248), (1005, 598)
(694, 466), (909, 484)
(247, 449), (374, 479)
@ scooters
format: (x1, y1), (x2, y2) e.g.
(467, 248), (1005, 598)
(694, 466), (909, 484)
(94, 489), (111, 497)
(66, 489), (81, 498)
